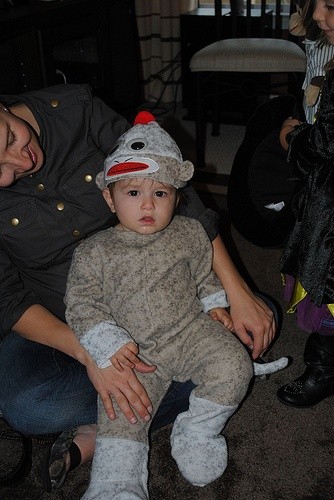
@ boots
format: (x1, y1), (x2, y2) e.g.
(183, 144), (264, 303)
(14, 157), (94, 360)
(276, 329), (333, 407)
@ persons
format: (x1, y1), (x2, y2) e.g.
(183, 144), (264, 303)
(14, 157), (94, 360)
(63, 112), (254, 500)
(278, 0), (334, 409)
(1, 83), (277, 492)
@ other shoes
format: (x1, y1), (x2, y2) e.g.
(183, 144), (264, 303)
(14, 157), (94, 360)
(46, 427), (84, 493)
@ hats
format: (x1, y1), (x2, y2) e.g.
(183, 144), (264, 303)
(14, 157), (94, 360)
(95, 114), (193, 188)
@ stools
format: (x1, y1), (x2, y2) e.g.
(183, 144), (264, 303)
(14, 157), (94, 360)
(188, 37), (306, 170)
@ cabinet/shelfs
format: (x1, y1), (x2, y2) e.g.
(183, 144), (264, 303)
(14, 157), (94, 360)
(180, 8), (273, 125)
(0, 22), (105, 103)
